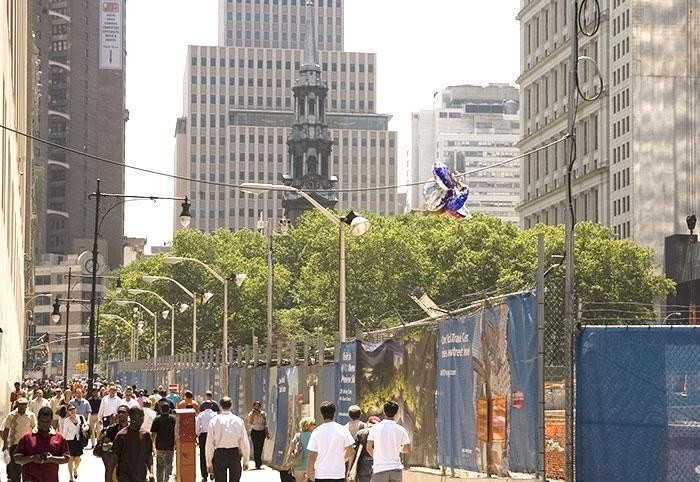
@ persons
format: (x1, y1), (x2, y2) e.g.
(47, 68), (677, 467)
(248, 401), (270, 470)
(0, 374), (250, 482)
(286, 401), (411, 482)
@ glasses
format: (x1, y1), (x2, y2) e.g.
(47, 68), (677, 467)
(118, 410), (126, 414)
(68, 407), (76, 411)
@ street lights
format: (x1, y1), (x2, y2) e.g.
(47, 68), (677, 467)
(99, 311), (143, 363)
(127, 288), (189, 371)
(26, 328), (83, 350)
(141, 275), (213, 364)
(257, 210), (291, 360)
(50, 296), (97, 401)
(26, 292), (53, 308)
(84, 176), (192, 401)
(163, 257), (247, 365)
(113, 297), (169, 369)
(238, 179), (371, 345)
(62, 266), (123, 394)
(662, 312), (682, 324)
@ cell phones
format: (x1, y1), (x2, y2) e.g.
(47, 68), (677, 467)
(39, 453), (50, 461)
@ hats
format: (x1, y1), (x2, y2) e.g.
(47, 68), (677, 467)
(16, 398), (28, 406)
(368, 416), (381, 424)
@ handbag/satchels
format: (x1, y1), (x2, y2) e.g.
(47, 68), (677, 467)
(79, 416), (89, 448)
(289, 432), (303, 466)
